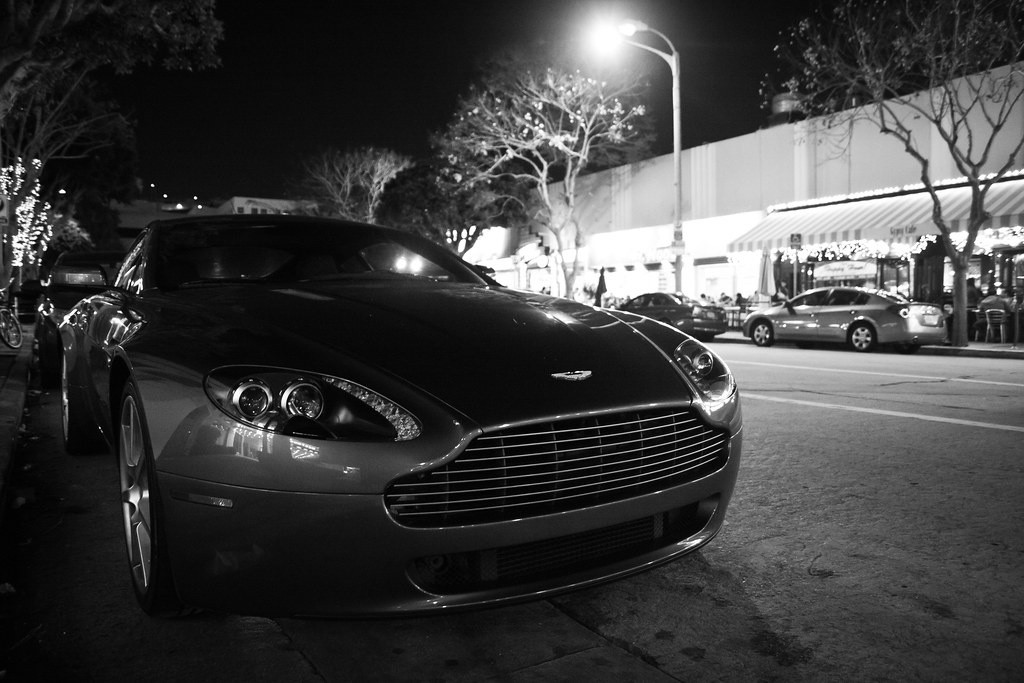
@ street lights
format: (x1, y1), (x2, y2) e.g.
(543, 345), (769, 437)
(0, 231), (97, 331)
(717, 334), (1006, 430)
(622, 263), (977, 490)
(571, 1), (684, 247)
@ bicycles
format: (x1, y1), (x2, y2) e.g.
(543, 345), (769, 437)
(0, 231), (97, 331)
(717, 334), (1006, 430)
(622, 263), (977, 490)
(0, 277), (23, 350)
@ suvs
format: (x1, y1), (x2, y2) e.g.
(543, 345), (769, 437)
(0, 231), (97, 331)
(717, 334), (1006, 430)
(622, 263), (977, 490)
(30, 248), (130, 395)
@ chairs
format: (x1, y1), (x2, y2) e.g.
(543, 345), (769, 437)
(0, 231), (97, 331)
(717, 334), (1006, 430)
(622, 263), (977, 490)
(985, 309), (1007, 342)
(159, 246), (231, 287)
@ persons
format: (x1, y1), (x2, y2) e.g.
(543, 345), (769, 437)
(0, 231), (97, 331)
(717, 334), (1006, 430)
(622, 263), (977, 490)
(536, 284), (786, 317)
(966, 277), (986, 341)
(999, 288), (1016, 310)
(973, 286), (1013, 340)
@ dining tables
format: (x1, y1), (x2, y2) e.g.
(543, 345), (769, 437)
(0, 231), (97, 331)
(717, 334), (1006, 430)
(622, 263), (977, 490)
(722, 307), (741, 329)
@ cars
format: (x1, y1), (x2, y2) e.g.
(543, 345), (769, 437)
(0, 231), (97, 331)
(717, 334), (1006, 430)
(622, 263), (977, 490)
(614, 292), (728, 342)
(20, 278), (44, 315)
(55, 214), (743, 620)
(743, 285), (948, 352)
